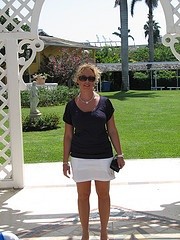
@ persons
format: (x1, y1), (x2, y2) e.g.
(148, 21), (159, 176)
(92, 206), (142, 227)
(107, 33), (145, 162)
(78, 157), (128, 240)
(62, 63), (125, 240)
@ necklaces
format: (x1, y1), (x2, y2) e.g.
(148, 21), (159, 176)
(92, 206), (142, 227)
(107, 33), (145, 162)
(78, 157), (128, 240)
(78, 93), (95, 104)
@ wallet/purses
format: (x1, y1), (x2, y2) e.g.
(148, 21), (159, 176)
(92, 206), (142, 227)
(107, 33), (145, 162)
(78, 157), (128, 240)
(110, 154), (120, 172)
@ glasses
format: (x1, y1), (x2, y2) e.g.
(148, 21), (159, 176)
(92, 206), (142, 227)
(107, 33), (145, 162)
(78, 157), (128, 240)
(79, 76), (95, 82)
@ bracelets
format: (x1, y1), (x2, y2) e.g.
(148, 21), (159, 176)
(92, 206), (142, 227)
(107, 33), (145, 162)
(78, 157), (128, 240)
(117, 154), (124, 158)
(63, 162), (69, 165)
